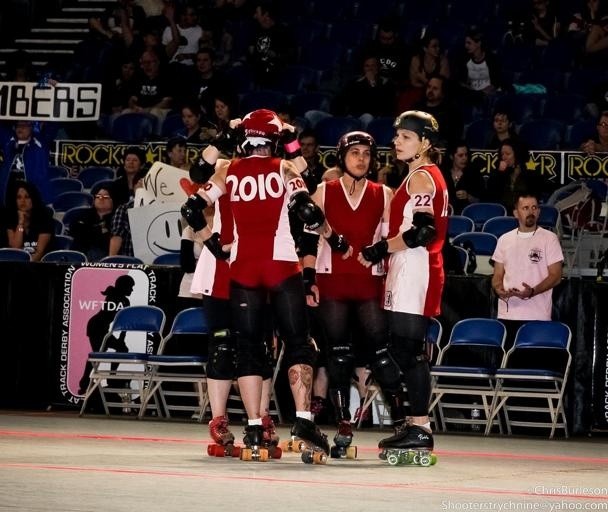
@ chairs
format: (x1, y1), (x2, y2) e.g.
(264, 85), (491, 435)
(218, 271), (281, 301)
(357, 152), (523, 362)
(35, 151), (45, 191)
(0, 1), (607, 438)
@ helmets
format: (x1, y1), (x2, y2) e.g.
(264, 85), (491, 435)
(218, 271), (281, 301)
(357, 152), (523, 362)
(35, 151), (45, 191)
(336, 132), (377, 159)
(393, 109), (440, 147)
(241, 108), (284, 136)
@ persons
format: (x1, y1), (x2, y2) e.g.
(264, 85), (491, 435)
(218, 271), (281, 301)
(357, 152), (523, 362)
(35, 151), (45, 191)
(180, 119), (309, 446)
(77, 274), (135, 396)
(177, 204), (213, 308)
(491, 191), (562, 435)
(189, 108), (354, 449)
(311, 131), (409, 449)
(0, 0), (608, 263)
(357, 109), (449, 450)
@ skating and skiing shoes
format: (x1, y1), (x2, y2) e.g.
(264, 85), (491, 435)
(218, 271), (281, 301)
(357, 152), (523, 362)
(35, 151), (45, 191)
(378, 422), (437, 466)
(207, 414), (282, 462)
(277, 417), (357, 464)
